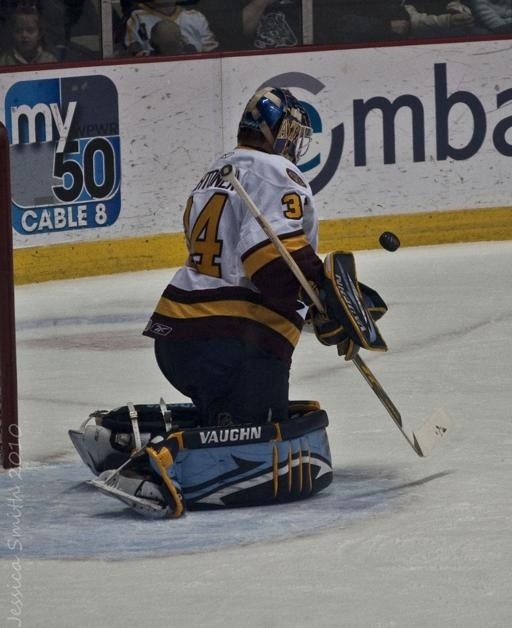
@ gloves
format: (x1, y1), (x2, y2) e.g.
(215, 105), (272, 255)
(298, 259), (360, 362)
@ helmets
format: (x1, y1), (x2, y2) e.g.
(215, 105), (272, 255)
(237, 88), (309, 166)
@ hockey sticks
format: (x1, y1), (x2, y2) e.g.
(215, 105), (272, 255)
(220, 164), (446, 457)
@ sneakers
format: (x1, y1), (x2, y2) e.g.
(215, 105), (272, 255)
(82, 408), (133, 475)
(98, 467), (186, 519)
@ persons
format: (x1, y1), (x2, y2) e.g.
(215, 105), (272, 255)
(0, 0), (512, 66)
(79, 82), (387, 519)
